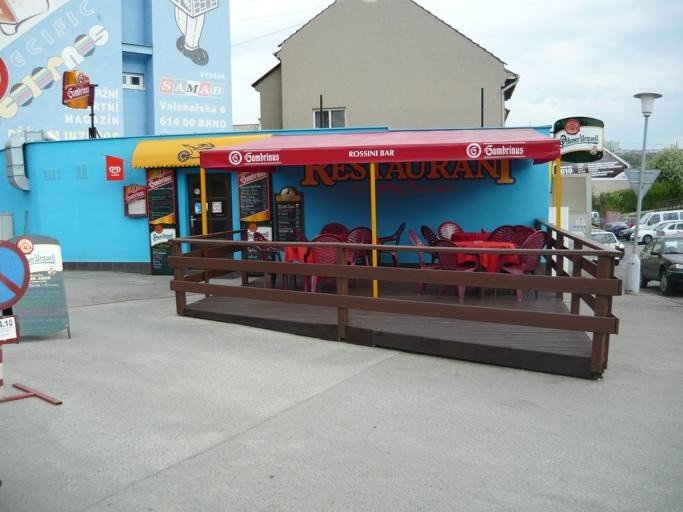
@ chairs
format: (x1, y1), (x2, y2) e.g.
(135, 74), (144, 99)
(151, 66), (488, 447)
(252, 221), (549, 302)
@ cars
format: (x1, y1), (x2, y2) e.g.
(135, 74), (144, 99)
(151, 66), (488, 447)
(591, 210), (683, 295)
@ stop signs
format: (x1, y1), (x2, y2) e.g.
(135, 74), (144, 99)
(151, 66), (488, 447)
(0, 239), (30, 311)
